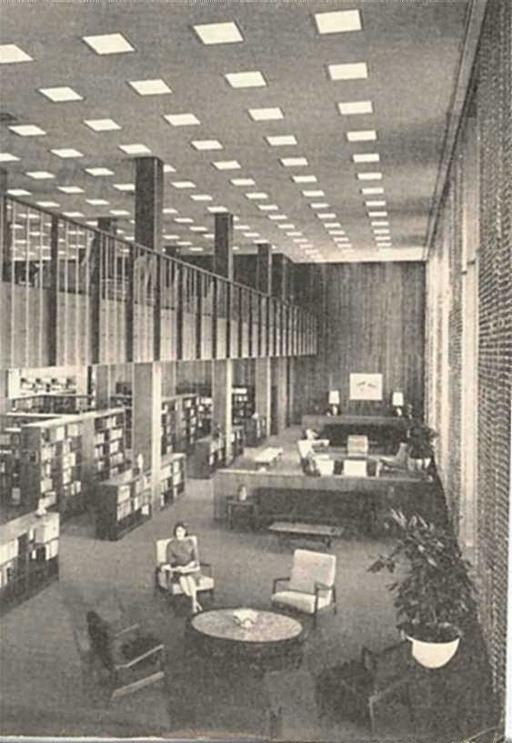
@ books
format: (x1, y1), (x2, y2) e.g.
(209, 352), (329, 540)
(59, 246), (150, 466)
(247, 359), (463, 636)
(163, 387), (264, 465)
(0, 378), (182, 588)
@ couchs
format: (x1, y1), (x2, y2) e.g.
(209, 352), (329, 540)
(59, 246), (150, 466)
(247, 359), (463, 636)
(252, 486), (390, 539)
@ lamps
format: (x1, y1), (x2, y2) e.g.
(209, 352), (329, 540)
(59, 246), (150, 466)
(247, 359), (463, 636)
(327, 390), (341, 415)
(391, 392), (406, 416)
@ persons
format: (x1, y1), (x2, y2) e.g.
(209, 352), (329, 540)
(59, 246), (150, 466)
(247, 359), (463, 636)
(165, 520), (202, 614)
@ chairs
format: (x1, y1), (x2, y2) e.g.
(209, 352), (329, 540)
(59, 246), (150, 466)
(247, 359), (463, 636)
(81, 611), (169, 712)
(272, 548), (339, 633)
(295, 423), (414, 479)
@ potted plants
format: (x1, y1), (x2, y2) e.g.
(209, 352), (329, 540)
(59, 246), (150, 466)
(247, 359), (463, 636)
(408, 423), (439, 470)
(369, 505), (479, 672)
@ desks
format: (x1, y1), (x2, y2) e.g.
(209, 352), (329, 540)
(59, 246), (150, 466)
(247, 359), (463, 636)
(323, 648), (417, 734)
(182, 608), (307, 682)
(266, 520), (346, 553)
(253, 447), (283, 470)
(225, 495), (259, 534)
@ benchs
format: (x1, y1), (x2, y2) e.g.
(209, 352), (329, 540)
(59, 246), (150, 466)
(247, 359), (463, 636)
(164, 700), (282, 741)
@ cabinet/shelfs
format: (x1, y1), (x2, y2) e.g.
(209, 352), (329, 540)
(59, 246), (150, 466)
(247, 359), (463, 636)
(0, 379), (278, 614)
